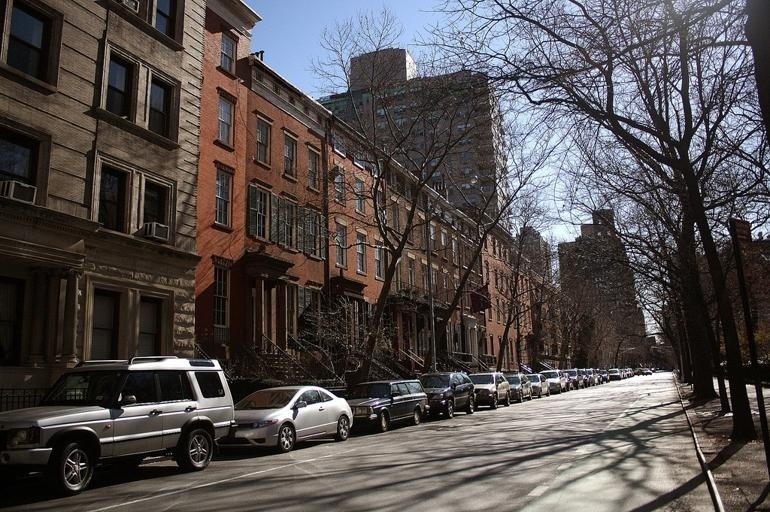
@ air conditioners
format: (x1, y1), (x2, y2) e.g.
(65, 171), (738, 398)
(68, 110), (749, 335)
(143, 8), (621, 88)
(119, 0), (140, 14)
(4, 181), (38, 205)
(143, 222), (170, 241)
(335, 166), (345, 175)
